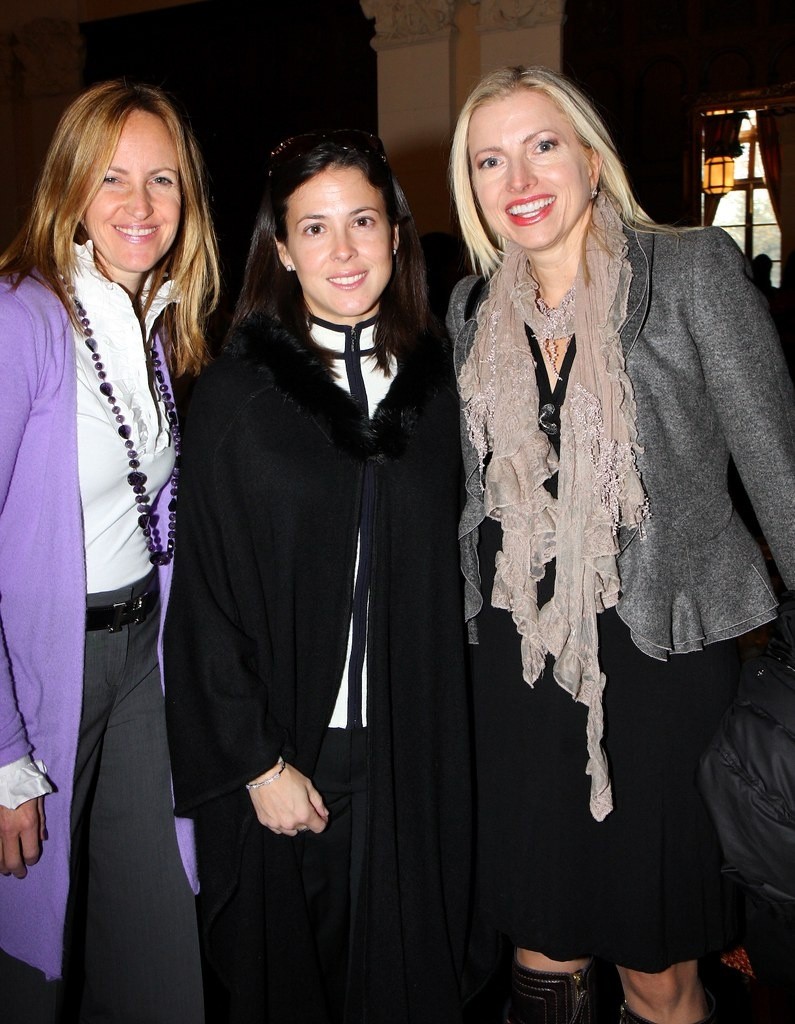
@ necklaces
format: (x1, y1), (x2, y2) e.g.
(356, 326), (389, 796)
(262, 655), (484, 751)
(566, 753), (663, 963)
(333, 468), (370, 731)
(51, 262), (185, 567)
(534, 272), (578, 390)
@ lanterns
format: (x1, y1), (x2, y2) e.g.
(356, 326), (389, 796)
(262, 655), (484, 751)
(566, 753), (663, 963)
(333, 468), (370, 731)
(699, 112), (750, 160)
(705, 108), (737, 115)
(701, 154), (736, 198)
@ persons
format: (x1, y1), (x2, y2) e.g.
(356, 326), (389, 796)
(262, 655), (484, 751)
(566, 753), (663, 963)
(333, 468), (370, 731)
(444, 62), (795, 1024)
(0, 80), (219, 1024)
(747, 254), (783, 325)
(174, 123), (511, 1022)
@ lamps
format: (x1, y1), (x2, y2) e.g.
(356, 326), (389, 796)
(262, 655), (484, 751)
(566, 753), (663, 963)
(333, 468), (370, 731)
(699, 108), (751, 194)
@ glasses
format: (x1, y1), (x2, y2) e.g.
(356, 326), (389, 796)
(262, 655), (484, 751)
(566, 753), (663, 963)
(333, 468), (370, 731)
(273, 127), (386, 168)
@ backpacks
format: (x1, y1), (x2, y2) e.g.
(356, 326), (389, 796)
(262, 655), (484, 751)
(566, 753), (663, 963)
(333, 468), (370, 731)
(700, 636), (793, 916)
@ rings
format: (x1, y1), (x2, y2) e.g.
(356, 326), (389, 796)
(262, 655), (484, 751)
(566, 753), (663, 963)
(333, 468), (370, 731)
(297, 827), (310, 831)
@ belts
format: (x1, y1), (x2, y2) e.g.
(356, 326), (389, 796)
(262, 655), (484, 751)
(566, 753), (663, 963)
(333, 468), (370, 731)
(84, 586), (160, 634)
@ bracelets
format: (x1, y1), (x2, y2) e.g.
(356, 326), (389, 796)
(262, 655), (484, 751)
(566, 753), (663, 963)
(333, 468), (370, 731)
(242, 759), (289, 788)
